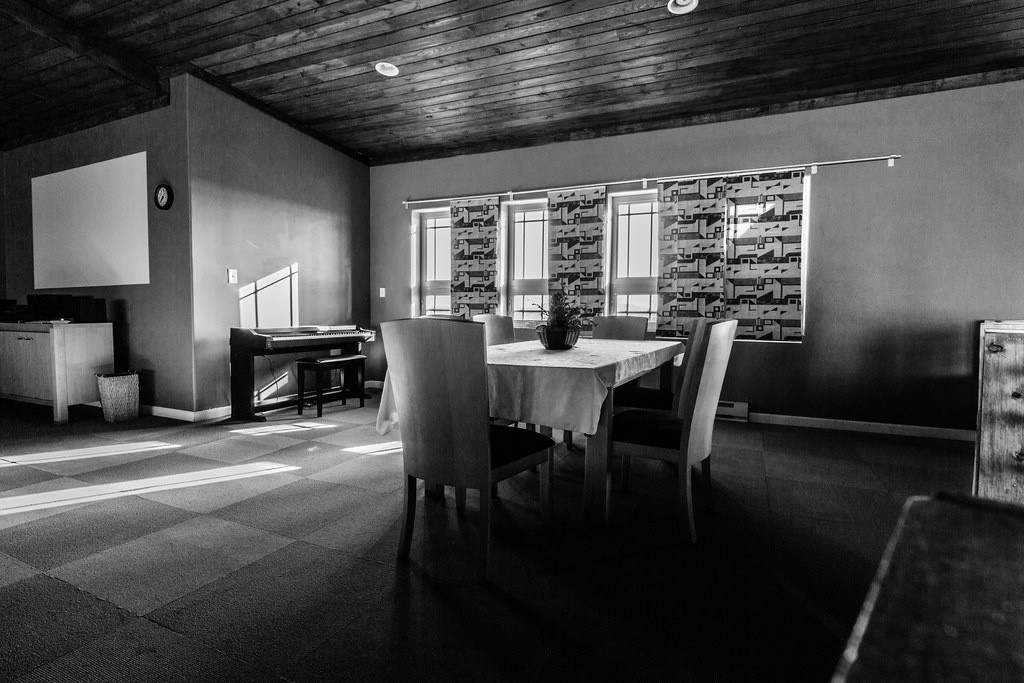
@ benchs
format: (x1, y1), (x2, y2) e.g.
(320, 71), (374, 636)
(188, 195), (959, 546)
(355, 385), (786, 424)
(296, 354), (367, 417)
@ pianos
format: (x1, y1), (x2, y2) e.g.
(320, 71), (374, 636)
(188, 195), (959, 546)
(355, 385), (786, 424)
(228, 323), (377, 421)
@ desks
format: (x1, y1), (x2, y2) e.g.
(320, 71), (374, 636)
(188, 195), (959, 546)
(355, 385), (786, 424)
(375, 337), (686, 527)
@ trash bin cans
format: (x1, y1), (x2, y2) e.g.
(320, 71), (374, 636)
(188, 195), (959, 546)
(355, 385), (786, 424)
(94, 368), (140, 423)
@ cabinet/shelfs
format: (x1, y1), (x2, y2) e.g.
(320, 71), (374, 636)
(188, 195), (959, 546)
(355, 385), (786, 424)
(977, 333), (1024, 506)
(0, 330), (53, 401)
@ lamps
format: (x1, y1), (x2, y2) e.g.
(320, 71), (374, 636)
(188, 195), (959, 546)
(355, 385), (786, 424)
(667, 0), (699, 15)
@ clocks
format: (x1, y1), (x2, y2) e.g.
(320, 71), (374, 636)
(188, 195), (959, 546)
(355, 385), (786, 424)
(154, 184), (174, 210)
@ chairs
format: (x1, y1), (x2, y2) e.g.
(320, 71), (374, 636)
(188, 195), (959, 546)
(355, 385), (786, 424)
(380, 312), (738, 581)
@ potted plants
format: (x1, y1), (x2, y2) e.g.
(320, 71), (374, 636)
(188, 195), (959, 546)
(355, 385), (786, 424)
(531, 283), (598, 349)
